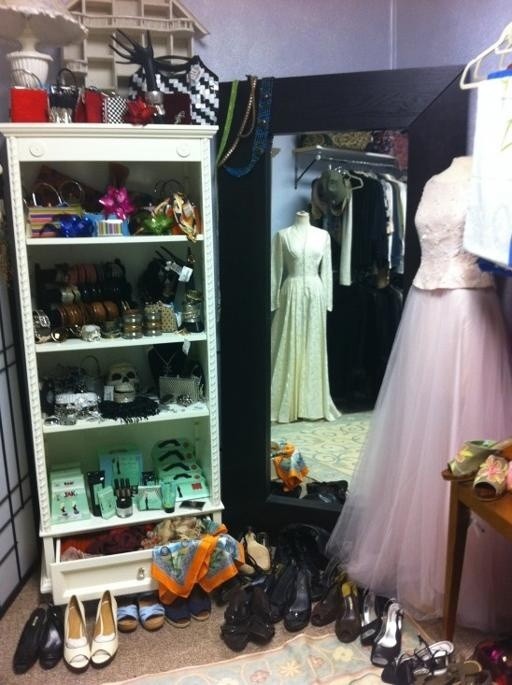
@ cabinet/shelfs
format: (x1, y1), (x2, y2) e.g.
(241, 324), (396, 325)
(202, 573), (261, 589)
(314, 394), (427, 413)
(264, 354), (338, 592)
(1, 116), (224, 614)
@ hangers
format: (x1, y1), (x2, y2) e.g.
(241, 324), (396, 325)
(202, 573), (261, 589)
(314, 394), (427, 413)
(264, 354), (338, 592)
(318, 155), (398, 190)
(458, 16), (512, 90)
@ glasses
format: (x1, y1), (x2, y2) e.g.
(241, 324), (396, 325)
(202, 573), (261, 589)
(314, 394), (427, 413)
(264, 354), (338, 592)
(161, 393), (191, 405)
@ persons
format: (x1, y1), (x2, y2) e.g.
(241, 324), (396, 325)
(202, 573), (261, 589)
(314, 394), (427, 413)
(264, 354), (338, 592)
(326, 156), (512, 620)
(271, 211), (341, 423)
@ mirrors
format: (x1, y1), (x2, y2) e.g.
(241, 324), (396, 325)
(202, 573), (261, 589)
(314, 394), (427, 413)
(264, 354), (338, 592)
(214, 62), (472, 552)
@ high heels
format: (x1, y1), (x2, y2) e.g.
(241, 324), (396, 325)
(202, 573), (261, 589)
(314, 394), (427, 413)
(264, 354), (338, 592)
(472, 454), (511, 502)
(441, 436), (512, 482)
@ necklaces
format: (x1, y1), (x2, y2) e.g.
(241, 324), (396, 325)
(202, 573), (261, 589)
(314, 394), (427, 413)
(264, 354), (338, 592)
(154, 348), (177, 377)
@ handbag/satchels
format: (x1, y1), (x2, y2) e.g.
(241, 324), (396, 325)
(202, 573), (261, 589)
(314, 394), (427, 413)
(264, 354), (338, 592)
(10, 85), (49, 122)
(128, 56), (221, 125)
(73, 89), (104, 123)
(102, 94), (140, 123)
(50, 84), (79, 122)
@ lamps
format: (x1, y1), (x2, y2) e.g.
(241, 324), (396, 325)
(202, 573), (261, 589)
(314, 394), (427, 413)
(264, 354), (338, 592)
(1, 0), (89, 89)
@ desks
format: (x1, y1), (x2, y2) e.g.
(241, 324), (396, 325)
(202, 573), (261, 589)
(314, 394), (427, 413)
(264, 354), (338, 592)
(440, 479), (512, 652)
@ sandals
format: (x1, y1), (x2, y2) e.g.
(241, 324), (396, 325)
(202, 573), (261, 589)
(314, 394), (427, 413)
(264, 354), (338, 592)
(136, 594), (165, 630)
(188, 583), (211, 621)
(117, 595), (139, 632)
(382, 635), (493, 685)
(163, 596), (192, 628)
(216, 524), (404, 667)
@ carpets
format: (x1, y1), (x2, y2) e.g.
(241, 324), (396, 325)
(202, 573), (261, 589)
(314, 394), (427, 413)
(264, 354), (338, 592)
(96, 600), (438, 685)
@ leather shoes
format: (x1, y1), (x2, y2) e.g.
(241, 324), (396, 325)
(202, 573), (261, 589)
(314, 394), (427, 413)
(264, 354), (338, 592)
(13, 607), (46, 674)
(62, 595), (91, 672)
(40, 606), (64, 669)
(91, 591), (118, 667)
